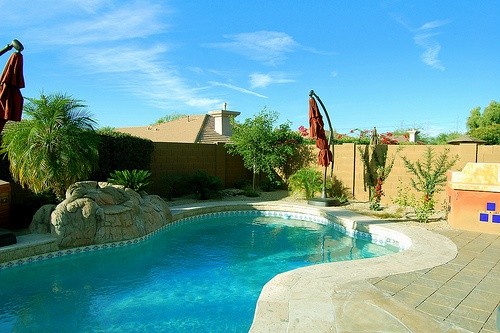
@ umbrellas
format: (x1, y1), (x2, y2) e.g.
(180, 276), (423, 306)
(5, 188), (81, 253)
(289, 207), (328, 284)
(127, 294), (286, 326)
(309, 96), (334, 166)
(0, 51), (26, 130)
